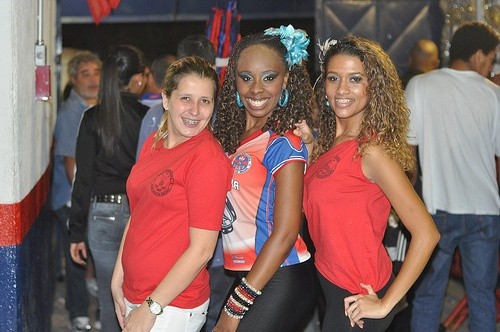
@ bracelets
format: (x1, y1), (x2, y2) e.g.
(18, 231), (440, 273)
(223, 277), (262, 319)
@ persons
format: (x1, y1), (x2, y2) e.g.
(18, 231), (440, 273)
(135, 34), (217, 163)
(197, 24), (320, 332)
(109, 55), (234, 332)
(402, 21), (500, 332)
(69, 45), (151, 332)
(305, 37), (441, 332)
(137, 54), (177, 108)
(399, 38), (440, 91)
(48, 50), (102, 332)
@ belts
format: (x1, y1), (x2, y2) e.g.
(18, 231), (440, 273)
(91, 194), (122, 204)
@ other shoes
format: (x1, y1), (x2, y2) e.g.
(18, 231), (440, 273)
(71, 315), (92, 332)
(93, 320), (102, 330)
(84, 277), (99, 297)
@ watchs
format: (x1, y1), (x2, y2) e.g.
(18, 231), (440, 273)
(145, 296), (163, 315)
